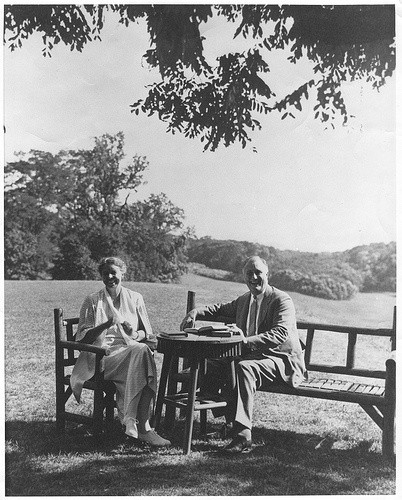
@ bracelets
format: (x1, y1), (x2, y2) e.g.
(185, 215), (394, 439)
(132, 331), (139, 341)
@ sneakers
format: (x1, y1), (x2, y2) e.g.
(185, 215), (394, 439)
(121, 416), (138, 438)
(137, 429), (171, 447)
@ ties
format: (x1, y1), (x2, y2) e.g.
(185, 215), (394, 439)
(248, 294), (258, 338)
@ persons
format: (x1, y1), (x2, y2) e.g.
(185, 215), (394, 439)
(70, 258), (171, 446)
(180, 256), (309, 454)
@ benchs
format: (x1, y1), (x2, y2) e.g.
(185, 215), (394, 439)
(183, 291), (396, 455)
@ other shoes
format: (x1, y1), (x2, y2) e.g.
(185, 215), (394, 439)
(225, 436), (252, 454)
(214, 423), (236, 441)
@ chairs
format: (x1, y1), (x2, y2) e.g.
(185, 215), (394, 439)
(54, 308), (117, 437)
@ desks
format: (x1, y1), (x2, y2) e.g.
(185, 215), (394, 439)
(156, 330), (243, 453)
